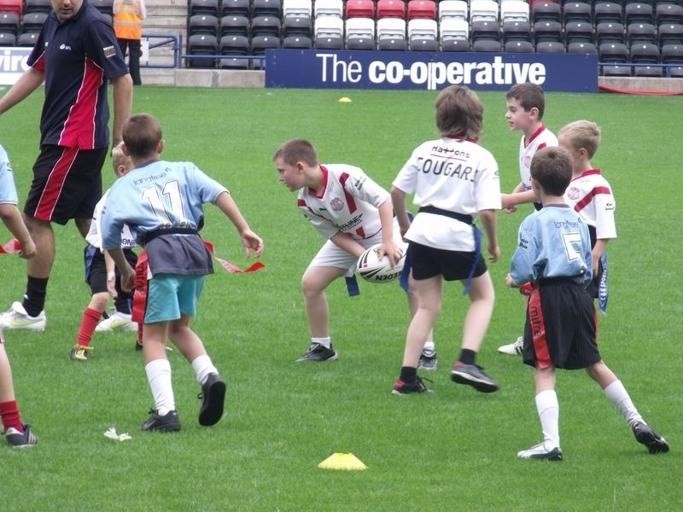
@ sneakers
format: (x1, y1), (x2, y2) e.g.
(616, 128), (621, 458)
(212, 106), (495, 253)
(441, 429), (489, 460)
(94, 312), (138, 333)
(295, 341), (339, 364)
(516, 443), (563, 461)
(142, 410), (181, 433)
(6, 423), (39, 449)
(391, 336), (524, 396)
(631, 423), (670, 453)
(2, 238), (24, 255)
(198, 372), (226, 425)
(0, 299), (47, 331)
(71, 342), (90, 360)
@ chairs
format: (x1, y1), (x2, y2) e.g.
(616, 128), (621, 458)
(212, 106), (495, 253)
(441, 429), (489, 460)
(185, 1), (683, 79)
(0, 0), (116, 46)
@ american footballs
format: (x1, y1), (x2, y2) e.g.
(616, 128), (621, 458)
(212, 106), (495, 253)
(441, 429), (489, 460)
(357, 244), (406, 282)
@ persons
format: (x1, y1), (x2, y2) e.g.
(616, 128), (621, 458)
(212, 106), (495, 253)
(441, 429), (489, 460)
(112, 0), (147, 86)
(69, 141), (172, 365)
(99, 114), (266, 433)
(501, 82), (561, 210)
(506, 146), (671, 463)
(273, 139), (439, 370)
(390, 84), (498, 396)
(496, 119), (616, 357)
(0, 0), (134, 331)
(0, 137), (39, 449)
